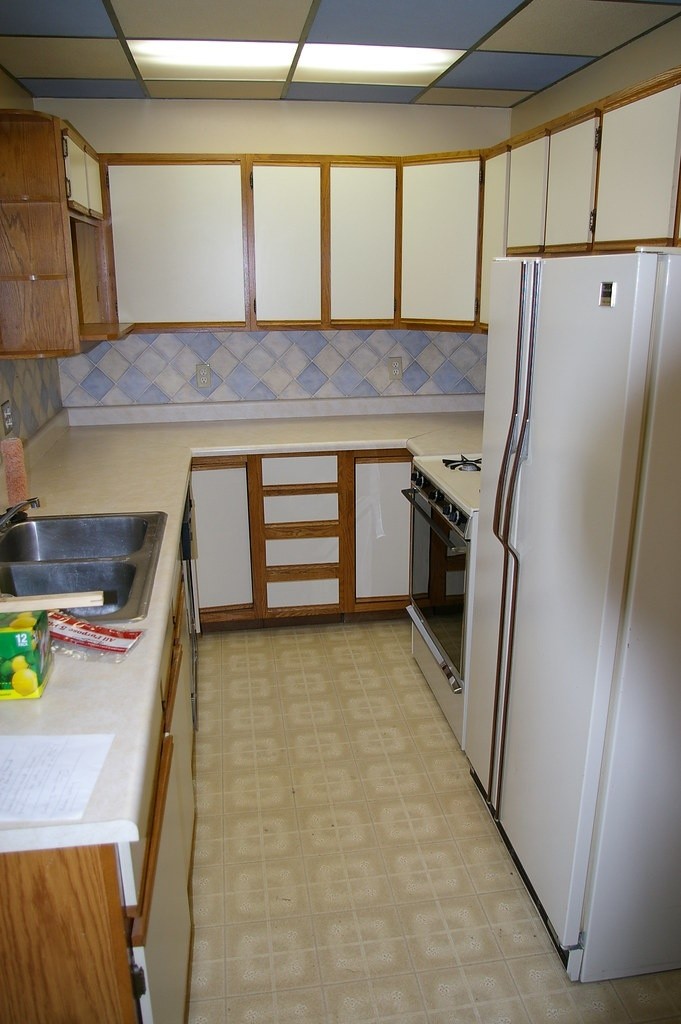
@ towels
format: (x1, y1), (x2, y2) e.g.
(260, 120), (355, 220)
(1, 437), (29, 512)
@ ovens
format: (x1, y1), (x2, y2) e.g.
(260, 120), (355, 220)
(404, 484), (477, 753)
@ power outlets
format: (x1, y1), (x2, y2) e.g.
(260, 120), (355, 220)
(1, 400), (13, 436)
(388, 357), (403, 380)
(197, 364), (211, 388)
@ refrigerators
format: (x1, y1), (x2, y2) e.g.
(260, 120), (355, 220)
(464, 251), (681, 986)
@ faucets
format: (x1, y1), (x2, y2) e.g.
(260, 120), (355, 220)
(0, 496), (41, 527)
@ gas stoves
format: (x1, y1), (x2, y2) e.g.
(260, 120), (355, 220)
(410, 454), (484, 540)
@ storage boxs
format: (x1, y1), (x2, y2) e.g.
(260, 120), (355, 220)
(0, 610), (54, 700)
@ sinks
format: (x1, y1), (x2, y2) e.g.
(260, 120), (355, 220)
(0, 510), (167, 561)
(0, 559), (155, 625)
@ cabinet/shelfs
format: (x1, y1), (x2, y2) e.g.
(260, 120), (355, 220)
(0, 448), (412, 1024)
(0, 66), (681, 359)
